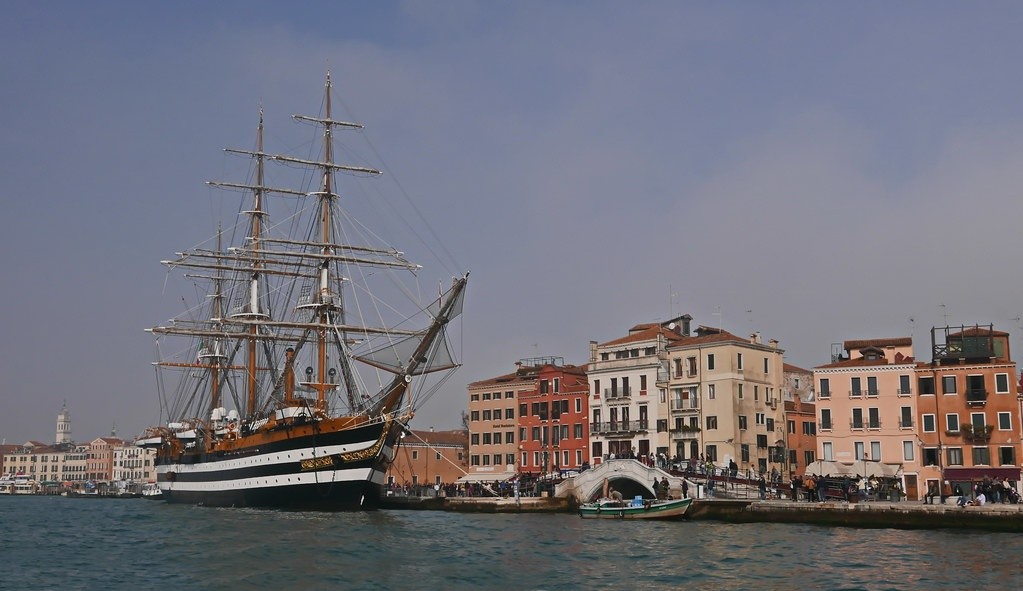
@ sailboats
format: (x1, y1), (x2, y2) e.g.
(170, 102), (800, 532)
(132, 69), (470, 509)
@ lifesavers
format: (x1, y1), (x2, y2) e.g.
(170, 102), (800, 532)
(229, 423), (235, 429)
(643, 503), (651, 512)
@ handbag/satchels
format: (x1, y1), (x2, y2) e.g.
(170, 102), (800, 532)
(790, 484), (793, 489)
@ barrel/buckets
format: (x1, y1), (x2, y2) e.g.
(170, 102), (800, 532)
(891, 490), (898, 501)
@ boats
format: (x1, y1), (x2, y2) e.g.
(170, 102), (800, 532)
(142, 481), (164, 499)
(579, 497), (692, 520)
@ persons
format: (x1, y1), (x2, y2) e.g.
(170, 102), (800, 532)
(578, 461), (590, 474)
(870, 477), (901, 494)
(680, 478), (688, 499)
(729, 458), (783, 482)
(923, 481), (937, 505)
(941, 474), (1019, 508)
(790, 474), (803, 502)
(385, 480), (415, 497)
(758, 475), (767, 500)
(518, 471), (537, 497)
(417, 482), (457, 498)
(652, 476), (670, 500)
(689, 453), (716, 475)
(610, 488), (624, 507)
(459, 480), (514, 497)
(708, 478), (713, 499)
(608, 449), (636, 459)
(637, 451), (682, 472)
(807, 474), (868, 502)
